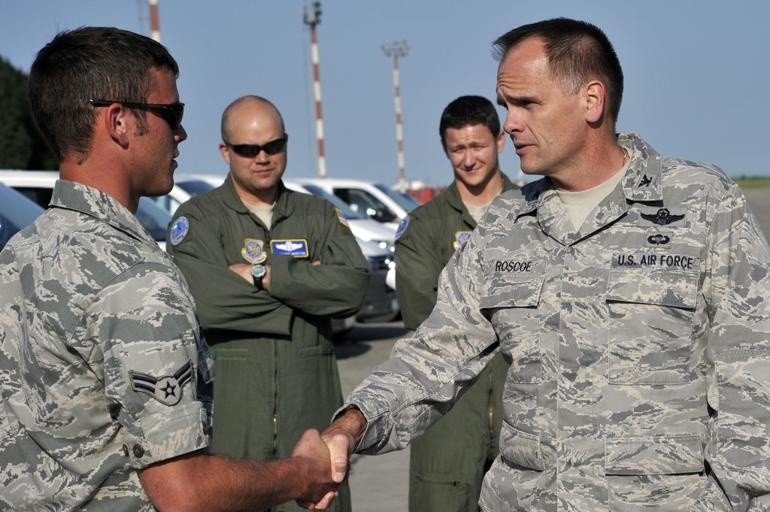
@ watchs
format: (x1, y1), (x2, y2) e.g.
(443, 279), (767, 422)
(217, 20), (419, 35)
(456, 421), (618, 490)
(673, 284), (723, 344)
(250, 264), (265, 288)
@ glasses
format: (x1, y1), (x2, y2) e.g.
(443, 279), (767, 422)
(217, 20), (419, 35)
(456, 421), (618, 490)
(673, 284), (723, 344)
(89, 100), (185, 129)
(225, 132), (288, 157)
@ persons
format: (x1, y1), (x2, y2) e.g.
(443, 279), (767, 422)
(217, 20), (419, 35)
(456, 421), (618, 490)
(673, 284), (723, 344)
(395, 95), (528, 512)
(165, 94), (372, 511)
(300, 16), (770, 512)
(0, 21), (341, 511)
